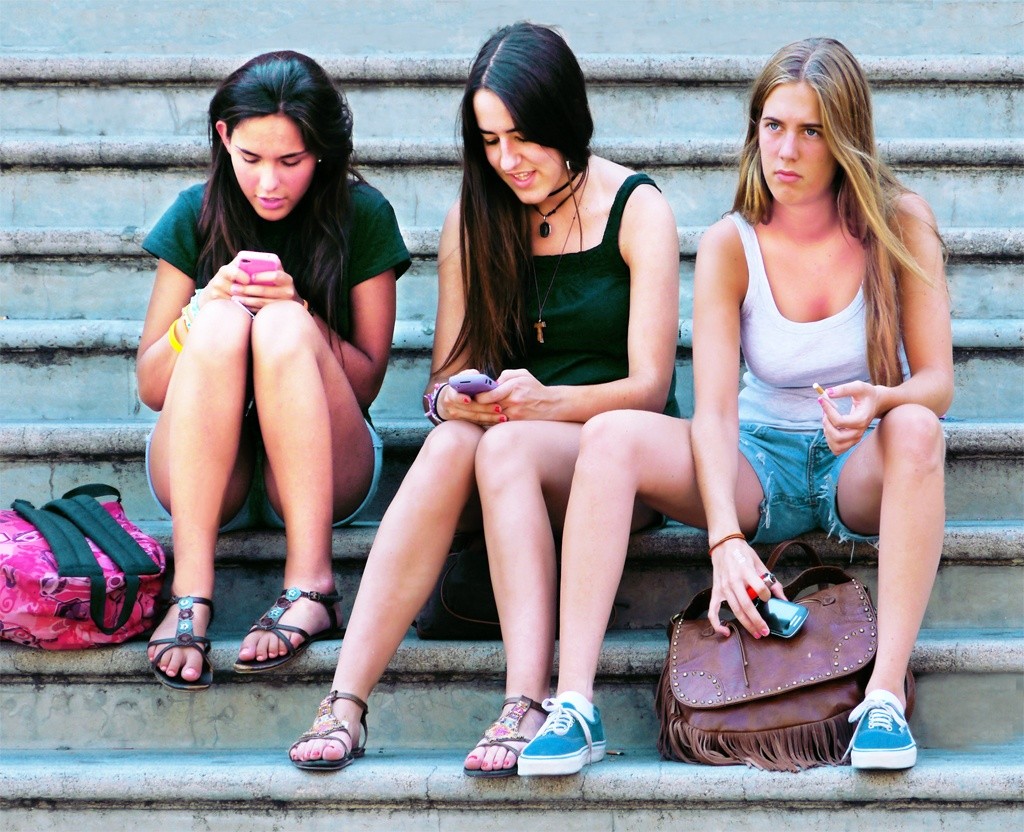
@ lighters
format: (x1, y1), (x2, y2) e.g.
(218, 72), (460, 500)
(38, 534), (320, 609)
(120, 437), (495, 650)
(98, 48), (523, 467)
(746, 573), (776, 601)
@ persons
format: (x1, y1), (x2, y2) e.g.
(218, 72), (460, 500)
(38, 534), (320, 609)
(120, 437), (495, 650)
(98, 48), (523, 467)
(137, 50), (410, 688)
(287, 23), (680, 778)
(518, 36), (953, 775)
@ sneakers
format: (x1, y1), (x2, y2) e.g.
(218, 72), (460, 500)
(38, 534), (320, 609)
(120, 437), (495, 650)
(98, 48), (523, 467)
(516, 696), (607, 777)
(840, 698), (918, 770)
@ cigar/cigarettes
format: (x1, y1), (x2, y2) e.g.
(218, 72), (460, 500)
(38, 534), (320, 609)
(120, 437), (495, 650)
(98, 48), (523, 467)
(813, 383), (838, 409)
(606, 751), (623, 756)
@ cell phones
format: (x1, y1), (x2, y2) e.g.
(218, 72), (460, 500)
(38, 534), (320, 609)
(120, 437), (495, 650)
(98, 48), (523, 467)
(232, 250), (280, 286)
(448, 373), (497, 395)
(753, 594), (810, 638)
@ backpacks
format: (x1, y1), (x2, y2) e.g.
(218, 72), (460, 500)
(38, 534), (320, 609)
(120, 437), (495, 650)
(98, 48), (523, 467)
(0, 483), (167, 652)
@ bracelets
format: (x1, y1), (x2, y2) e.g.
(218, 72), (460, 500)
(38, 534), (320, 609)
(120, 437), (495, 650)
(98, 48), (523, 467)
(168, 320), (183, 353)
(304, 300), (308, 310)
(708, 533), (745, 554)
(308, 304), (314, 316)
(182, 304), (191, 328)
(423, 382), (448, 424)
(183, 319), (188, 335)
(190, 289), (202, 318)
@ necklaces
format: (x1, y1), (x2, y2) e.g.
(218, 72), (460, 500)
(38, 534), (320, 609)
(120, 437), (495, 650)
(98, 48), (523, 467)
(530, 163), (590, 343)
(533, 168), (586, 238)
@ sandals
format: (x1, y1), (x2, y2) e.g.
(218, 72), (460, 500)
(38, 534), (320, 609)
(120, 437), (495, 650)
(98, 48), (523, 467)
(147, 591), (215, 692)
(288, 691), (369, 772)
(464, 696), (550, 779)
(232, 584), (344, 673)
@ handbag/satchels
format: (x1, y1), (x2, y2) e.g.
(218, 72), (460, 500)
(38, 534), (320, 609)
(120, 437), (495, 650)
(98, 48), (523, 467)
(650, 540), (917, 774)
(404, 530), (621, 643)
